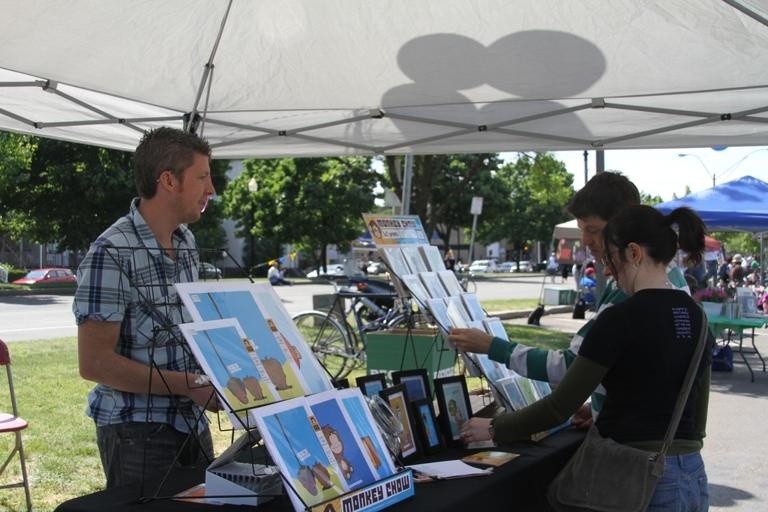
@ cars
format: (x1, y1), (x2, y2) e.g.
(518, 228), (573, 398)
(11, 267), (75, 287)
(308, 260), (388, 278)
(455, 259), (548, 274)
(198, 263), (224, 279)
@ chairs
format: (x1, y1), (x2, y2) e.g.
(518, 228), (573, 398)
(1, 340), (33, 512)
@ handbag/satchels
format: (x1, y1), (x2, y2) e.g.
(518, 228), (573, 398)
(546, 423), (666, 512)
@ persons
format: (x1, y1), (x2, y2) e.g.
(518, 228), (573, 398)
(542, 250), (600, 312)
(68, 124), (226, 500)
(708, 251), (768, 314)
(267, 261), (295, 286)
(462, 202), (717, 511)
(445, 248), (455, 270)
(445, 170), (691, 431)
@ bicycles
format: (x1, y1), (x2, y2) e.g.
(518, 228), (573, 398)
(291, 285), (468, 385)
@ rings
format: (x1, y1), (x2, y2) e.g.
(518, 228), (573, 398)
(453, 340), (457, 348)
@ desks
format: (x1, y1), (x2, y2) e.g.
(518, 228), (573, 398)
(53, 384), (589, 511)
(707, 315), (767, 384)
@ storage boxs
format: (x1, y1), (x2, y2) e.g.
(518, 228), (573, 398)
(544, 288), (581, 306)
(365, 327), (455, 400)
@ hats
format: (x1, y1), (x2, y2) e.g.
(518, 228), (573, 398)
(732, 254), (743, 262)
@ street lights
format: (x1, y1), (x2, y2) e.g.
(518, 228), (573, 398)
(675, 146), (767, 189)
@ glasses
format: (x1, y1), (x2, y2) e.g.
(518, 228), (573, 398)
(600, 250), (621, 266)
